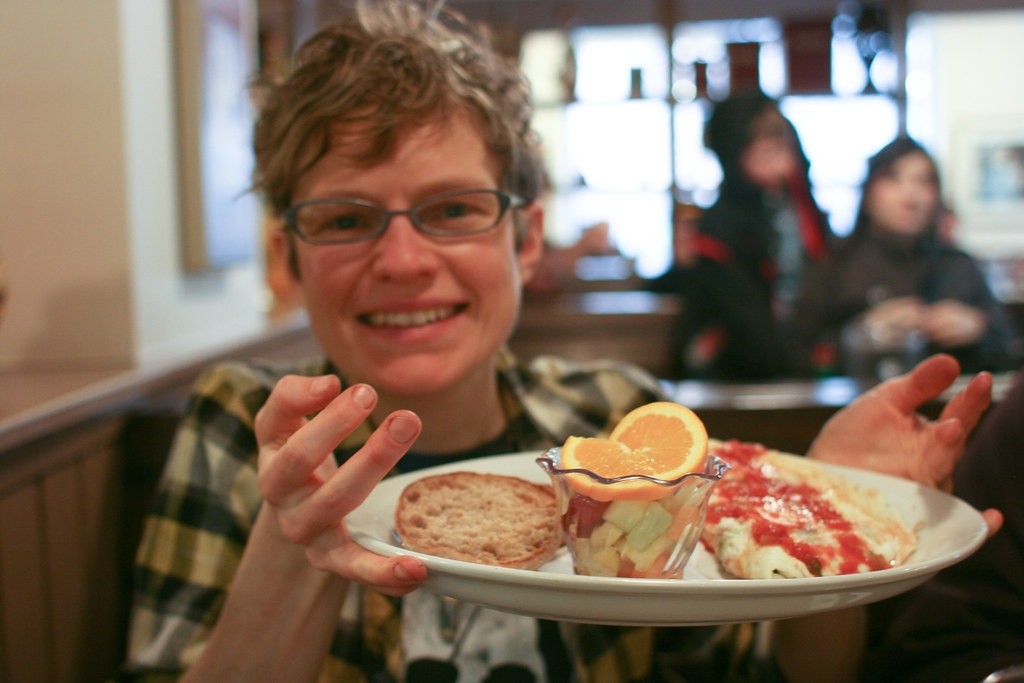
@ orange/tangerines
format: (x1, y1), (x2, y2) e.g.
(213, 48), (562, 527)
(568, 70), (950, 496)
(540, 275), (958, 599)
(560, 402), (709, 501)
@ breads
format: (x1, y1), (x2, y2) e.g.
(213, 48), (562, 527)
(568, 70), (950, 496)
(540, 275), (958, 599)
(394, 471), (562, 569)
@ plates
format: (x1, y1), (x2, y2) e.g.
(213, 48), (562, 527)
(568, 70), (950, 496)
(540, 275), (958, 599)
(341, 451), (988, 627)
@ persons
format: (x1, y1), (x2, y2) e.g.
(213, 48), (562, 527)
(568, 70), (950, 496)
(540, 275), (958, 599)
(871, 374), (1024, 683)
(649, 88), (1018, 379)
(125, 5), (1003, 682)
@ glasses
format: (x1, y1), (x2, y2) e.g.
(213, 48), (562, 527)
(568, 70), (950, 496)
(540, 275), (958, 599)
(278, 187), (511, 246)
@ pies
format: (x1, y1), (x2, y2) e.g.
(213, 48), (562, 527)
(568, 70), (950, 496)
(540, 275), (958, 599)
(695, 439), (918, 580)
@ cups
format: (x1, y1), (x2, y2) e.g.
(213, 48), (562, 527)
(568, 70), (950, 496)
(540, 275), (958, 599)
(538, 445), (731, 582)
